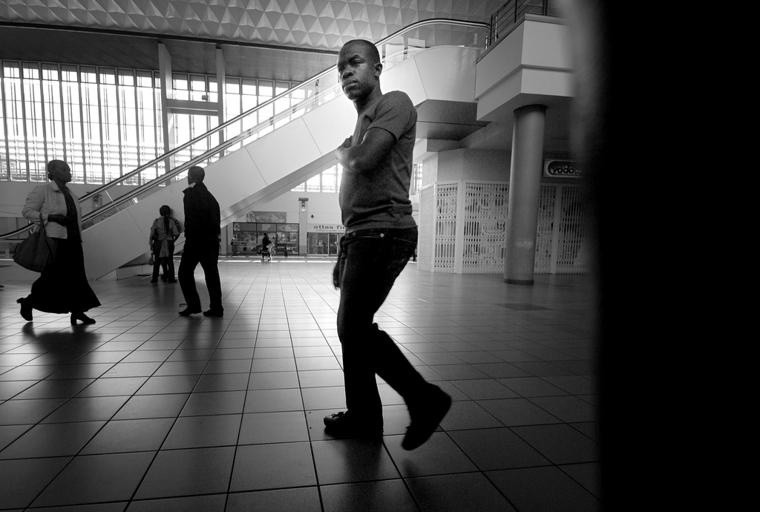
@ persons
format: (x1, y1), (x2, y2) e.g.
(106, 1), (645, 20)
(150, 204), (179, 284)
(178, 167), (224, 318)
(323, 39), (452, 452)
(159, 208), (182, 282)
(553, 1), (760, 511)
(261, 232), (273, 261)
(16, 159), (101, 326)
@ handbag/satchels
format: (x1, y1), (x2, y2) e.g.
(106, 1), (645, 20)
(13, 213), (64, 272)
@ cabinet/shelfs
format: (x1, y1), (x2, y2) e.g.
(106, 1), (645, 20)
(232, 221), (300, 256)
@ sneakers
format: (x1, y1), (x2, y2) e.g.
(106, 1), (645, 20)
(203, 309), (223, 318)
(323, 411), (384, 437)
(400, 388), (452, 450)
(178, 307), (202, 317)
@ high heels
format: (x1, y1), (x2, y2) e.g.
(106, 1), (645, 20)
(16, 297), (33, 321)
(71, 313), (95, 326)
